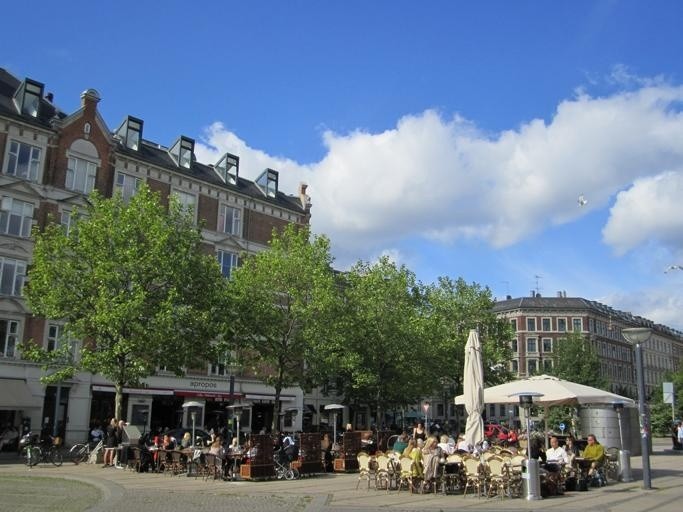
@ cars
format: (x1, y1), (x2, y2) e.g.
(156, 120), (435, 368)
(155, 426), (212, 444)
(484, 423), (518, 444)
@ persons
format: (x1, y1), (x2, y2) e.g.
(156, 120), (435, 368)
(672, 420), (683, 450)
(91, 419), (604, 497)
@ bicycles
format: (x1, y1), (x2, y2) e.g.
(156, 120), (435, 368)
(22, 433), (36, 471)
(68, 440), (91, 466)
(18, 433), (66, 467)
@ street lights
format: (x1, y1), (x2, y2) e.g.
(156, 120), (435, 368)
(422, 403), (429, 437)
(507, 410), (513, 420)
(224, 364), (241, 407)
(618, 325), (656, 489)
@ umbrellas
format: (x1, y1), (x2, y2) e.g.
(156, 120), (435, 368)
(463, 328), (485, 448)
(454, 373), (635, 450)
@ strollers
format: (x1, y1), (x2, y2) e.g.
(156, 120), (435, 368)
(271, 444), (302, 481)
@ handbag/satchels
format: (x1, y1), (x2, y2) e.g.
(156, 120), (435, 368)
(566, 477), (588, 490)
(591, 474), (607, 487)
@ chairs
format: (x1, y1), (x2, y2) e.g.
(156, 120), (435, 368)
(102, 424), (621, 502)
